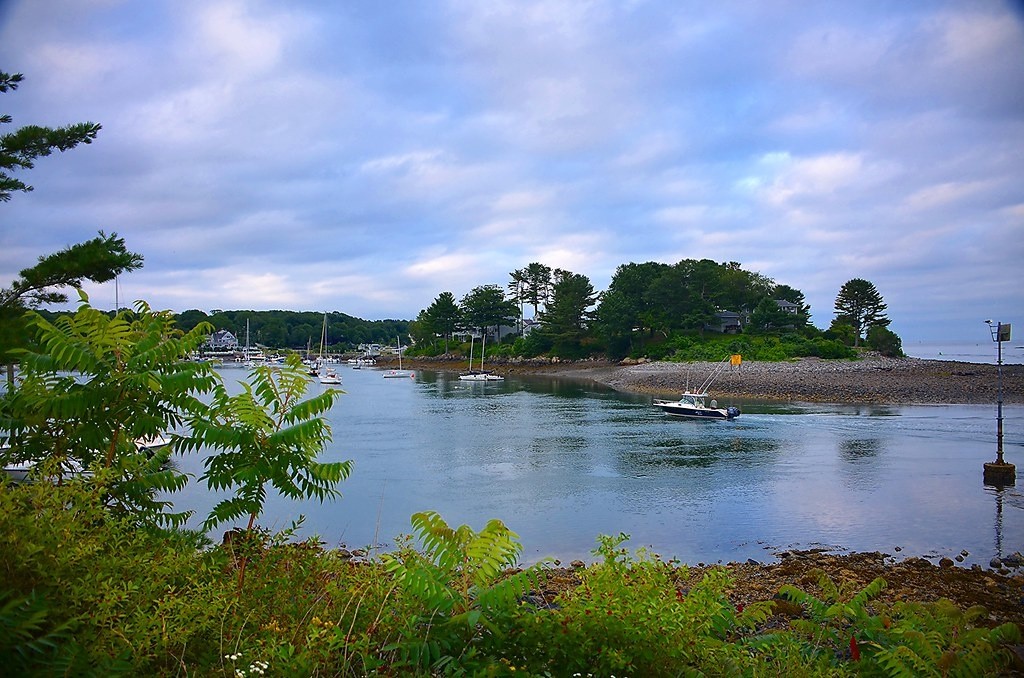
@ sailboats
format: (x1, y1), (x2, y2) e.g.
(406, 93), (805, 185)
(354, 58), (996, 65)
(381, 337), (413, 378)
(239, 319), (253, 367)
(459, 333), (504, 381)
(316, 311), (341, 383)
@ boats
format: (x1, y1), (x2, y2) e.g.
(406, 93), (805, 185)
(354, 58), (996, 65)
(383, 372), (412, 377)
(654, 355), (741, 417)
(307, 361), (319, 374)
